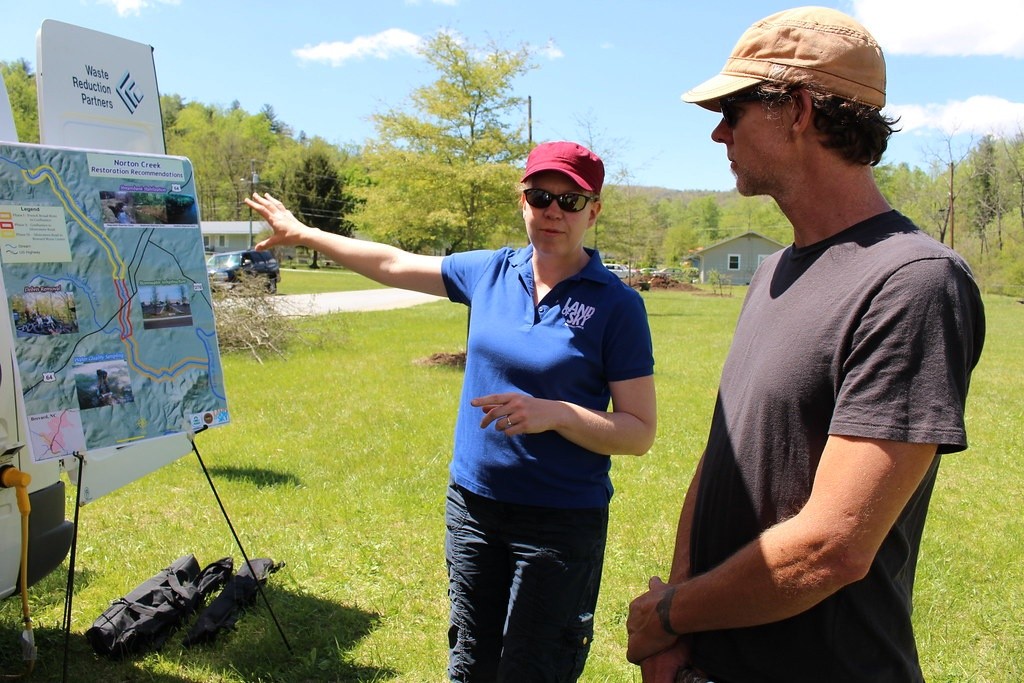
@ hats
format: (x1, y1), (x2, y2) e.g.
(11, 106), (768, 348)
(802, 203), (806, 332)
(519, 141), (605, 194)
(681, 6), (888, 110)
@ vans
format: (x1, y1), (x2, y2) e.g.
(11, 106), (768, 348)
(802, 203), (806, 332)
(601, 263), (636, 278)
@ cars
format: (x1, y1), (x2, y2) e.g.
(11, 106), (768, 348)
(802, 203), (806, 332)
(639, 267), (656, 274)
(649, 268), (685, 277)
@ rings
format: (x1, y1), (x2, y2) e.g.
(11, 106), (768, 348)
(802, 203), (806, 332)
(507, 416), (511, 426)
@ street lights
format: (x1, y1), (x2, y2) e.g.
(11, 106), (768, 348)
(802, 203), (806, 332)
(248, 157), (260, 251)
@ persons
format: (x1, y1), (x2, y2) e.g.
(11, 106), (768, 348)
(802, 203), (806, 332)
(12, 308), (20, 325)
(625, 4), (988, 683)
(240, 141), (656, 682)
(114, 203), (134, 224)
(25, 307), (37, 320)
(96, 369), (111, 395)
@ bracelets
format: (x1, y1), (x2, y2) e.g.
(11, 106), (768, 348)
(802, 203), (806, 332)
(655, 587), (679, 635)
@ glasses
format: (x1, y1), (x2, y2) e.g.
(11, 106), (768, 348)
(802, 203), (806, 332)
(523, 188), (599, 212)
(718, 95), (773, 128)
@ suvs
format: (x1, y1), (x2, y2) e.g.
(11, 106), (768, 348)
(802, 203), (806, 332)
(207, 249), (282, 294)
